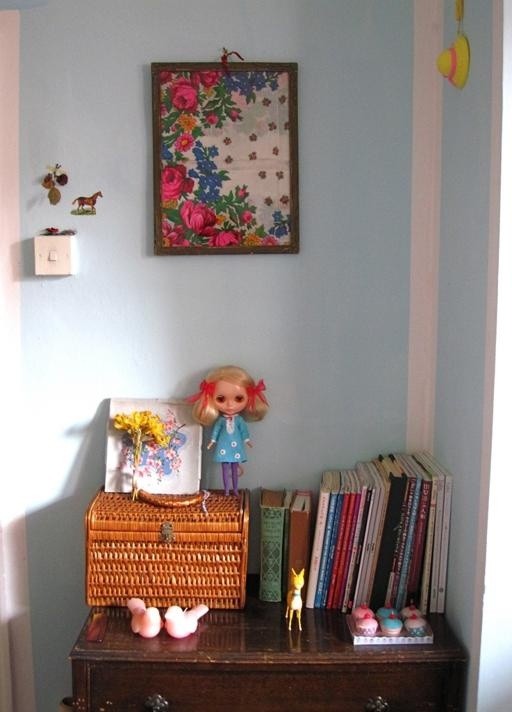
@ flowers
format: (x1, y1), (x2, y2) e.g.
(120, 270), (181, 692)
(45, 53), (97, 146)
(112, 408), (173, 505)
(157, 69), (292, 247)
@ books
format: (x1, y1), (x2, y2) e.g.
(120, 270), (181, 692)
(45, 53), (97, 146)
(257, 448), (455, 614)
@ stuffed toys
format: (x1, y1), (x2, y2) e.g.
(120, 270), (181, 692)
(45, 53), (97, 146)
(164, 603), (209, 639)
(127, 596), (163, 638)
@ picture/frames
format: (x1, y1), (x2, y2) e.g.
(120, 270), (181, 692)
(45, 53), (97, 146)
(149, 60), (300, 256)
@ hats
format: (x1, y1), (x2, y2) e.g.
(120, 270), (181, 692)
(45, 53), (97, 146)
(436, 33), (472, 90)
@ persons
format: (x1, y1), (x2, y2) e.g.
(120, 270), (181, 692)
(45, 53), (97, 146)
(192, 363), (269, 498)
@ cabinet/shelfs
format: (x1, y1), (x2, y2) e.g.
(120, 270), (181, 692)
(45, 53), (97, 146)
(67, 565), (471, 711)
